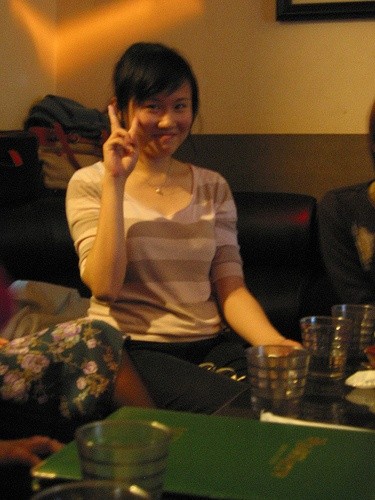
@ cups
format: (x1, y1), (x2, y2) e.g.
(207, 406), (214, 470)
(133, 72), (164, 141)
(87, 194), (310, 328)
(331, 303), (374, 368)
(244, 345), (312, 417)
(33, 479), (151, 500)
(301, 316), (350, 380)
(76, 421), (173, 498)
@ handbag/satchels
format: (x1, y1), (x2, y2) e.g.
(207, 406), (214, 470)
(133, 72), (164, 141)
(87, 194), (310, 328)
(27, 94), (111, 171)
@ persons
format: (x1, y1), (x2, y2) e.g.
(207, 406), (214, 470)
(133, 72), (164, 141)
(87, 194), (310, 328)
(0, 278), (154, 468)
(318, 100), (375, 305)
(65, 42), (305, 414)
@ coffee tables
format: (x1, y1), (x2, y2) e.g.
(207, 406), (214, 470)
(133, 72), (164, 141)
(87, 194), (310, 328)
(211, 354), (375, 433)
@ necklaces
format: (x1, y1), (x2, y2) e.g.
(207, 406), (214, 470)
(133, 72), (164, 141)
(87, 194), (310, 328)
(135, 160), (174, 193)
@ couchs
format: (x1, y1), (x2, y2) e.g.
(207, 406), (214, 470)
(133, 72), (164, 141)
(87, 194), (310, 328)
(0, 190), (315, 344)
